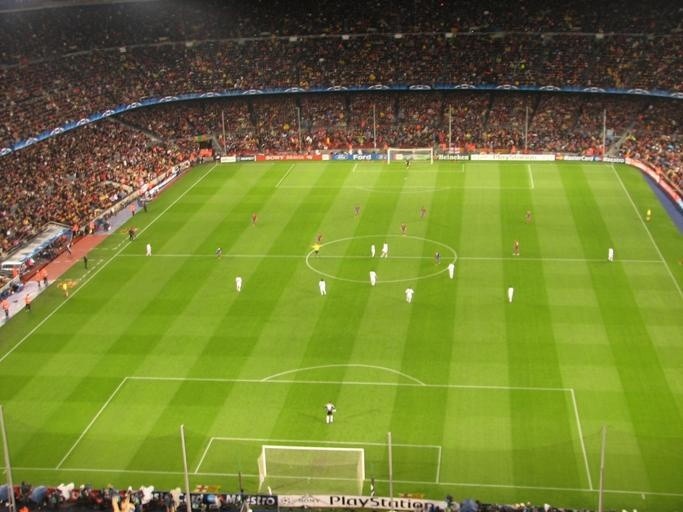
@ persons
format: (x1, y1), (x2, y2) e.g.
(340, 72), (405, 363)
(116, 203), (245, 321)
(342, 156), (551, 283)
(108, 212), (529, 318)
(353, 203), (360, 220)
(511, 239), (520, 256)
(0, 482), (558, 512)
(251, 211), (256, 225)
(317, 278), (325, 295)
(507, 285), (513, 302)
(434, 251), (440, 263)
(525, 208), (531, 224)
(607, 244), (613, 262)
(236, 275), (242, 291)
(399, 222), (406, 237)
(369, 269), (379, 286)
(420, 205), (425, 219)
(0, 162), (191, 319)
(447, 261), (454, 278)
(645, 209), (652, 223)
(316, 231), (322, 242)
(324, 400), (334, 423)
(215, 247), (221, 261)
(404, 286), (414, 304)
(370, 241), (376, 257)
(2, 1), (680, 197)
(380, 241), (388, 258)
(312, 242), (321, 256)
